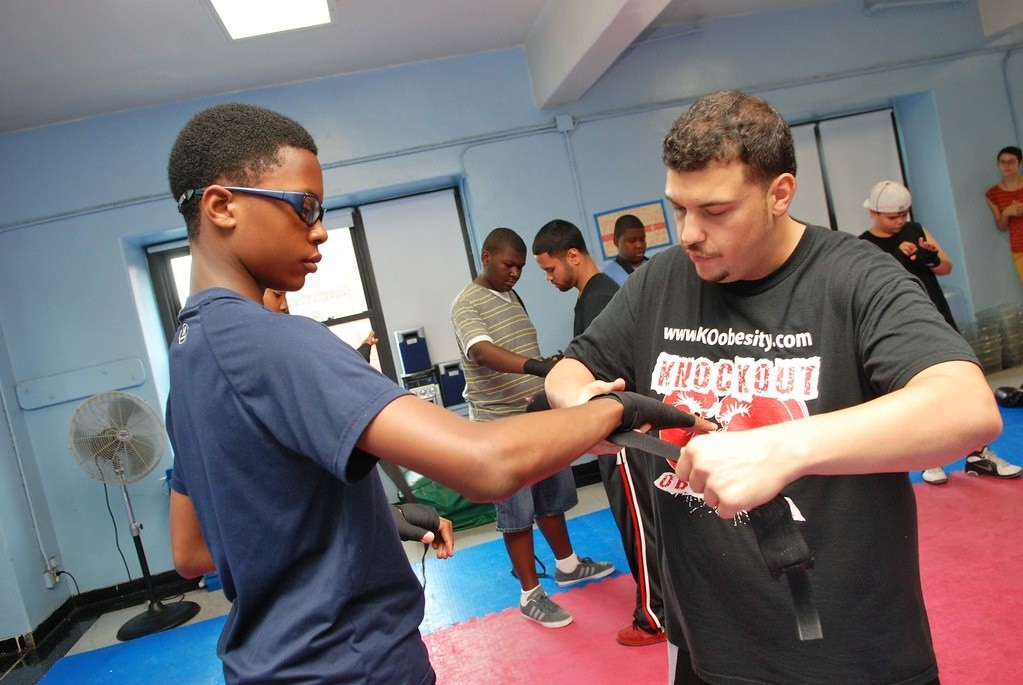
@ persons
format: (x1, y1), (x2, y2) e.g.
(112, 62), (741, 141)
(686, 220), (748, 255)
(985, 146), (1023, 283)
(452, 227), (616, 628)
(263, 288), (379, 366)
(858, 181), (1022, 486)
(532, 219), (667, 647)
(544, 89), (1004, 685)
(165, 104), (718, 685)
(602, 214), (651, 287)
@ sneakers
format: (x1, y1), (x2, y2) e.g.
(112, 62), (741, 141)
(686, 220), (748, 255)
(615, 622), (666, 647)
(922, 467), (947, 484)
(519, 585), (573, 628)
(964, 445), (1021, 478)
(554, 554), (615, 586)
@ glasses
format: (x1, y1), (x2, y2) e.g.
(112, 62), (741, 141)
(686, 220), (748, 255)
(997, 158), (1018, 165)
(176, 185), (328, 228)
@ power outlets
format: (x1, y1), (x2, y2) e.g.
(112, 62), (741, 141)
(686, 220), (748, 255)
(43, 567), (62, 589)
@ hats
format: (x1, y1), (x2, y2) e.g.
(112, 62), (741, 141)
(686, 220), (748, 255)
(864, 179), (912, 213)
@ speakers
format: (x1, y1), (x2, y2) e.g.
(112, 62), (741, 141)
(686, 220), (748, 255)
(394, 326), (432, 375)
(401, 366), (444, 409)
(435, 359), (466, 407)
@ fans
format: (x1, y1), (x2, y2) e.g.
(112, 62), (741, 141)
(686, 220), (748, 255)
(66, 390), (202, 641)
